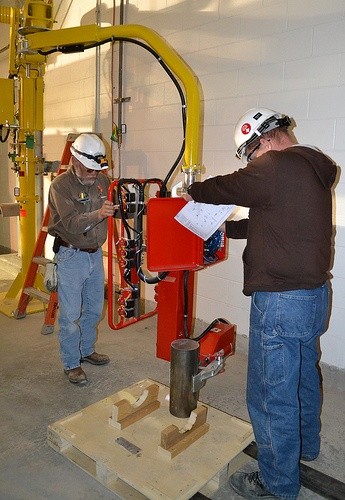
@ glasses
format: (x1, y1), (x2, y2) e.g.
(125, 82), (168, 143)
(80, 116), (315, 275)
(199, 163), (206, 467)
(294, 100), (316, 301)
(86, 169), (102, 173)
(246, 140), (271, 163)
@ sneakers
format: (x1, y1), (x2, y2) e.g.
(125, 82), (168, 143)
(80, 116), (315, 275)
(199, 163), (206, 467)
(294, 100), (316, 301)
(228, 470), (281, 500)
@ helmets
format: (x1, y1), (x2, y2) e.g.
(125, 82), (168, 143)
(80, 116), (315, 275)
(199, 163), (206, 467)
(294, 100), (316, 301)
(70, 133), (109, 170)
(232, 106), (292, 160)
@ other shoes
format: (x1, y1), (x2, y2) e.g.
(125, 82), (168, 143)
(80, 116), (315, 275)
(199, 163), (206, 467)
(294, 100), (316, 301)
(80, 352), (110, 365)
(64, 366), (87, 385)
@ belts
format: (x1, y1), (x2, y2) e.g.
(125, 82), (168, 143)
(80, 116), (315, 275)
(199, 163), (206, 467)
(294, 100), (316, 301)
(60, 240), (98, 254)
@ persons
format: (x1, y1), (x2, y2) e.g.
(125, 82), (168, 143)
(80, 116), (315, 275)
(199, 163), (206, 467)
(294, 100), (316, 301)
(177, 108), (337, 500)
(48, 134), (147, 384)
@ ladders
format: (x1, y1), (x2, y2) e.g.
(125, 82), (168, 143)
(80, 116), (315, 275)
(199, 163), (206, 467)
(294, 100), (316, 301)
(12, 131), (103, 336)
